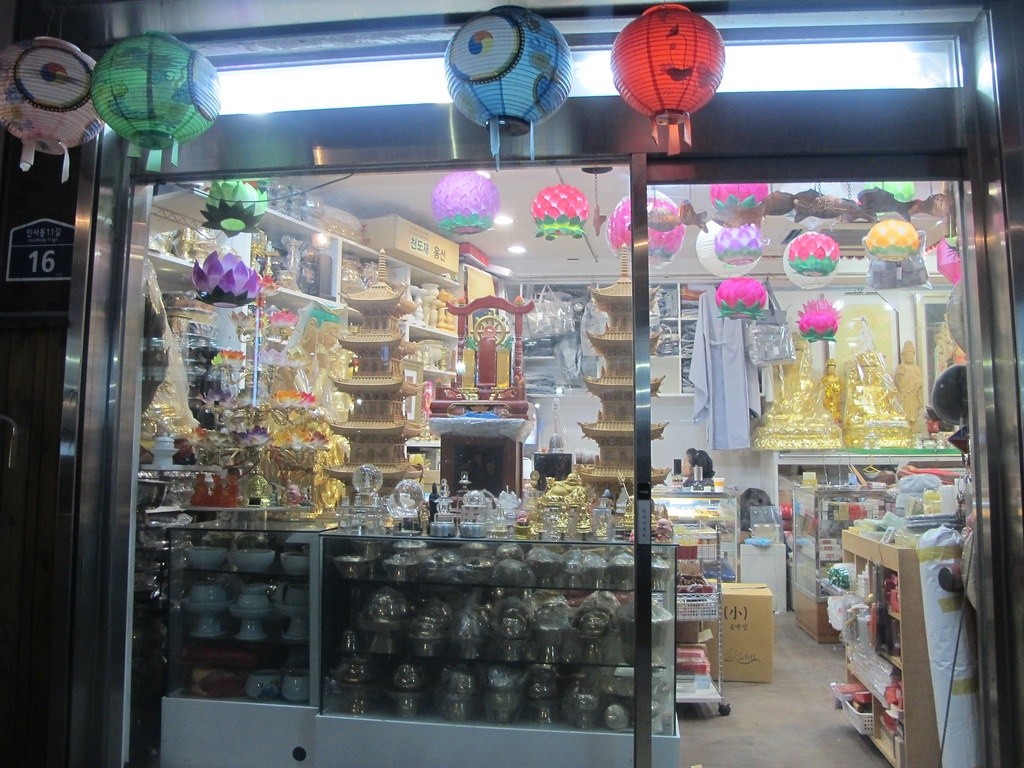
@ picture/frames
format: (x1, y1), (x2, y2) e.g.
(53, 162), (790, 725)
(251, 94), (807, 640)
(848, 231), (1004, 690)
(914, 290), (968, 417)
(822, 286), (902, 376)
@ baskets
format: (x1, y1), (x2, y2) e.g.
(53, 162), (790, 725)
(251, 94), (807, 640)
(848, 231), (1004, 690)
(677, 596), (719, 621)
(843, 701), (874, 734)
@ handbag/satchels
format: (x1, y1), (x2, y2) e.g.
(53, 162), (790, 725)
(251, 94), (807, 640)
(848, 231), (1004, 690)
(525, 284), (573, 341)
(746, 280), (797, 367)
(860, 230), (928, 289)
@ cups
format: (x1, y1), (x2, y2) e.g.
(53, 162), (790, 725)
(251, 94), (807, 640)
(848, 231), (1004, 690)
(714, 478), (725, 491)
(154, 433), (175, 448)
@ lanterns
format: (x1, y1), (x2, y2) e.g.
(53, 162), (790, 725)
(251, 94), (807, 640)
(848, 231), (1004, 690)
(199, 179), (267, 238)
(443, 7), (573, 136)
(192, 251), (263, 308)
(90, 28), (224, 149)
(0, 30), (106, 154)
(608, 4), (727, 126)
(430, 166), (965, 345)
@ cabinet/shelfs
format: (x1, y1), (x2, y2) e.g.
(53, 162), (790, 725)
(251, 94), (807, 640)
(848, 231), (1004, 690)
(739, 544), (787, 614)
(169, 520), (676, 733)
(151, 180), (511, 450)
(761, 447), (966, 543)
(514, 279), (702, 396)
(841, 528), (941, 768)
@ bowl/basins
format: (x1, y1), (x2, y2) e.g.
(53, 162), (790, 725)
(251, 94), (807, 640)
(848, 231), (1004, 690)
(185, 546), (317, 574)
(244, 668), (310, 702)
(190, 582), (226, 603)
(284, 582), (310, 606)
(235, 595), (268, 609)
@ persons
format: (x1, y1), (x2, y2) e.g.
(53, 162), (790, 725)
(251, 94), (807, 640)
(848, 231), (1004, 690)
(839, 349), (909, 423)
(682, 447), (716, 489)
(893, 340), (924, 435)
(822, 358), (842, 426)
(516, 471), (609, 538)
(655, 517), (672, 541)
(767, 330), (829, 418)
(270, 301), (351, 518)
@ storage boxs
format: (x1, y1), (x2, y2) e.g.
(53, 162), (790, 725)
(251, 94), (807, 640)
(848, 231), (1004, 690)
(701, 580), (774, 682)
(363, 215), (459, 273)
(676, 622), (714, 643)
